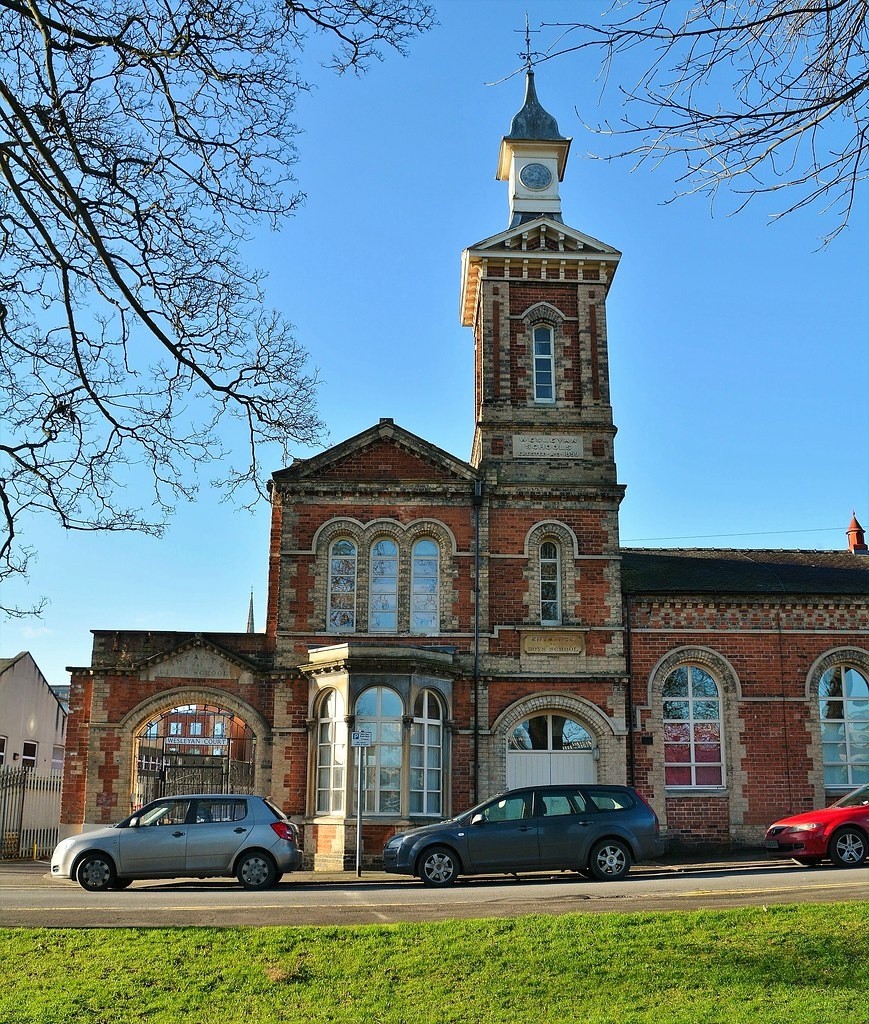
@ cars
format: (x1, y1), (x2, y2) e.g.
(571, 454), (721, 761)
(50, 794), (304, 892)
(765, 784), (869, 869)
(382, 783), (665, 888)
